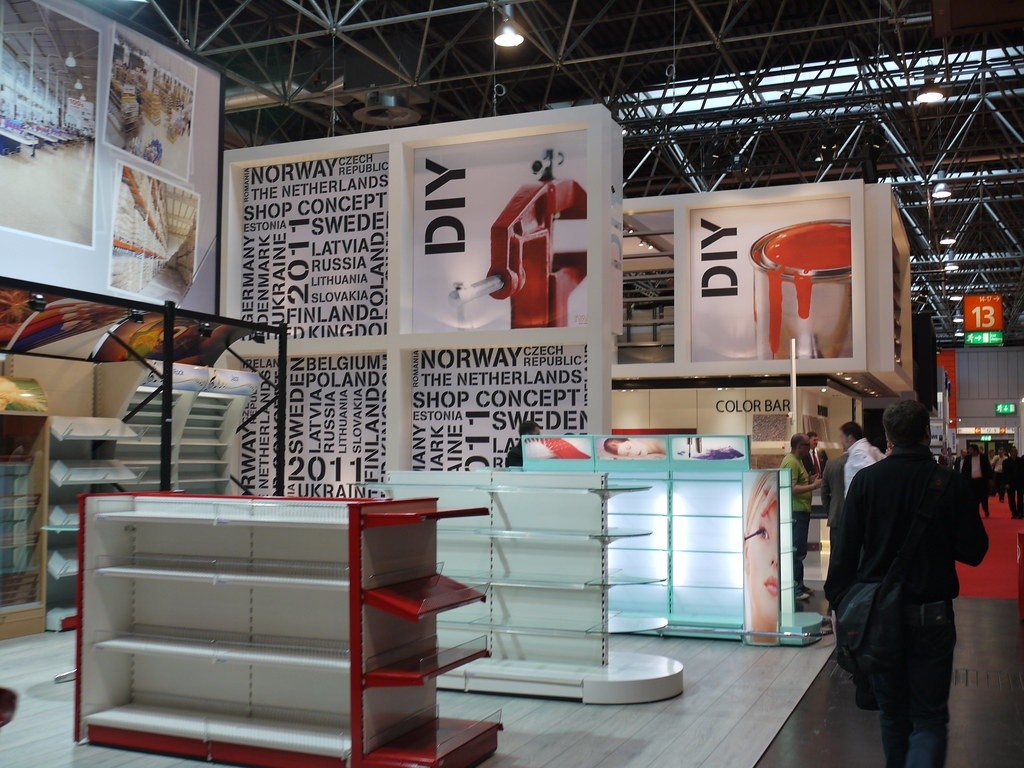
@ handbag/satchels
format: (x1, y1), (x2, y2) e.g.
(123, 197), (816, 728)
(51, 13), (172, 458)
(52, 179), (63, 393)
(837, 582), (907, 678)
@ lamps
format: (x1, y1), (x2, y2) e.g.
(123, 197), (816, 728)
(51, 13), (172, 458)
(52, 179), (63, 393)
(27, 292), (47, 312)
(494, 4), (524, 47)
(932, 171), (952, 199)
(955, 324), (964, 337)
(945, 249), (959, 271)
(866, 134), (891, 150)
(128, 308), (143, 324)
(949, 285), (963, 301)
(821, 132), (838, 149)
(940, 223), (956, 245)
(916, 66), (943, 102)
(953, 310), (965, 323)
(198, 322), (212, 338)
(254, 332), (266, 344)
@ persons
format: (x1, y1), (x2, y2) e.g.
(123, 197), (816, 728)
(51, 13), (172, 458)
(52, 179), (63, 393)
(779, 433), (822, 599)
(505, 421), (540, 467)
(939, 444), (1024, 519)
(821, 421), (893, 630)
(113, 62), (192, 135)
(743, 471), (780, 644)
(604, 438), (666, 456)
(824, 399), (989, 768)
(802, 431), (829, 478)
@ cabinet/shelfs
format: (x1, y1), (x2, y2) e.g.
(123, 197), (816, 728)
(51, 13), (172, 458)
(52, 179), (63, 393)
(0, 349), (825, 768)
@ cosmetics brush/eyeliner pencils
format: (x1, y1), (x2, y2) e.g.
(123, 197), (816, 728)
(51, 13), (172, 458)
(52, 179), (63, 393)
(745, 528), (764, 540)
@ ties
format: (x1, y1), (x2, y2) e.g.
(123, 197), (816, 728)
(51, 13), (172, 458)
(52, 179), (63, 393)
(812, 450), (819, 473)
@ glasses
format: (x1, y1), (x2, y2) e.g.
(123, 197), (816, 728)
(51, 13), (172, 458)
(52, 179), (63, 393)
(800, 442), (810, 447)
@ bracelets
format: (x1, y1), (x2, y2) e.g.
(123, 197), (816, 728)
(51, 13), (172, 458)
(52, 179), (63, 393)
(812, 483), (817, 489)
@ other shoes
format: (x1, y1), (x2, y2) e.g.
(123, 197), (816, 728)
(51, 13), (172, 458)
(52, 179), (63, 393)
(1011, 513), (1023, 519)
(795, 588), (814, 600)
